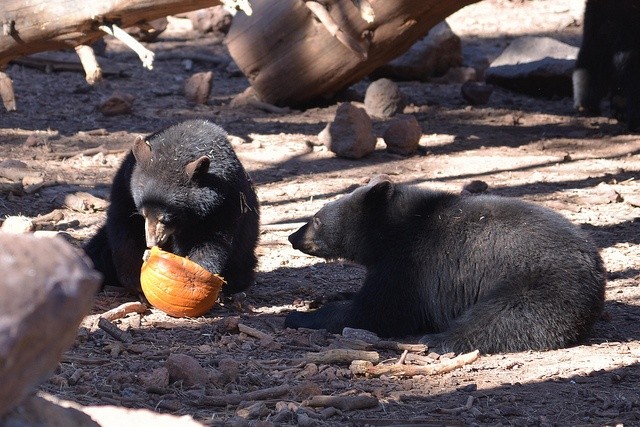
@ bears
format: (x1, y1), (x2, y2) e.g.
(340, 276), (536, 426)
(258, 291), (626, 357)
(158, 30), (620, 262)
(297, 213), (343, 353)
(286, 173), (607, 354)
(84, 120), (260, 294)
